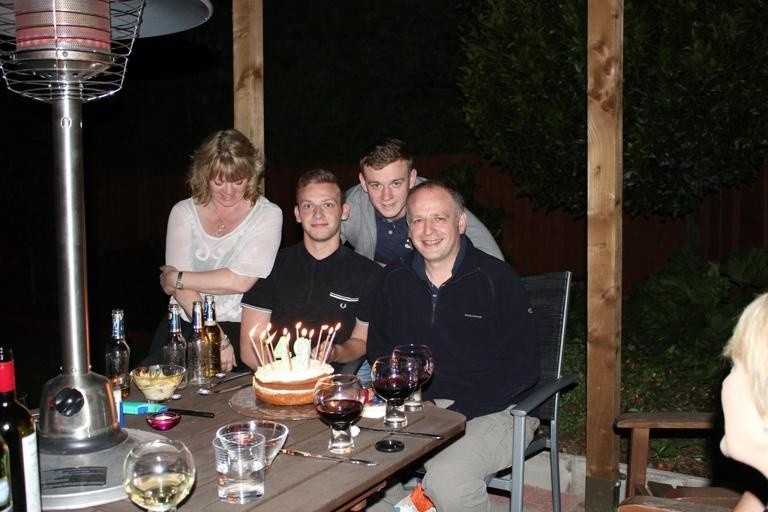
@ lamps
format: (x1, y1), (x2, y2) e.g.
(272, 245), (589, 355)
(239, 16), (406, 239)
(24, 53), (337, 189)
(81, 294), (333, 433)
(0, 0), (215, 511)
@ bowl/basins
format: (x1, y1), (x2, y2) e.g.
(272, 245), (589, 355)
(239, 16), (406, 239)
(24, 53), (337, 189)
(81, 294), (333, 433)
(218, 420), (288, 469)
(130, 364), (186, 406)
(148, 413), (182, 431)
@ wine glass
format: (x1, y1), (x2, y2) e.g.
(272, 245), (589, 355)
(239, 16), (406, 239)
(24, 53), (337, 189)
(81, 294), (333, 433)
(314, 344), (435, 454)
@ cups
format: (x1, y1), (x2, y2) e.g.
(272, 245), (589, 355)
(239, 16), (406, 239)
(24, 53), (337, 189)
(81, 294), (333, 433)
(123, 440), (196, 512)
(214, 433), (265, 503)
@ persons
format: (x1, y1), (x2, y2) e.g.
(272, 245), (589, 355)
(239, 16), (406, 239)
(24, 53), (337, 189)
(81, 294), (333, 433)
(140, 128), (285, 370)
(365, 181), (540, 512)
(337, 137), (507, 403)
(720, 291), (768, 512)
(238, 169), (378, 371)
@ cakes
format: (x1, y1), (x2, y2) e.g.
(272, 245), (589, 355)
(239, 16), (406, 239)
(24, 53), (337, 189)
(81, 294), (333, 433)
(252, 356), (335, 407)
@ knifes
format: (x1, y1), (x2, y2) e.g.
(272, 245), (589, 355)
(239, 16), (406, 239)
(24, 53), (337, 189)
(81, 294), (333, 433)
(359, 425), (443, 441)
(281, 448), (379, 467)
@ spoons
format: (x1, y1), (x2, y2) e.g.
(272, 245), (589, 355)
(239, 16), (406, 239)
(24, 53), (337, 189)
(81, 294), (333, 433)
(196, 383), (252, 394)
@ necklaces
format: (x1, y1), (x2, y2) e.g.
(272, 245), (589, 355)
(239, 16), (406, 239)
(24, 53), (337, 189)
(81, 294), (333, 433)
(208, 199), (243, 238)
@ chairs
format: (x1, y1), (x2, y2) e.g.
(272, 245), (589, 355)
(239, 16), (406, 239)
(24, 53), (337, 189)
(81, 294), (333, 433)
(614, 412), (742, 512)
(415, 271), (579, 512)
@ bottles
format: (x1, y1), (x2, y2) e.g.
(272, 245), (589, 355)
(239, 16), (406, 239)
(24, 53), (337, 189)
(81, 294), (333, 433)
(164, 296), (221, 388)
(106, 309), (130, 401)
(0, 346), (42, 511)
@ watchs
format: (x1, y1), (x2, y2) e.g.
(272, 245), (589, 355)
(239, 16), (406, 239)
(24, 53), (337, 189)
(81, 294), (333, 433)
(176, 272), (183, 290)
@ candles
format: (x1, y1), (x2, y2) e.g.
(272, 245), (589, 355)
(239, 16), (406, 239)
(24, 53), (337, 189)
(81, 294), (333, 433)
(248, 321), (342, 370)
